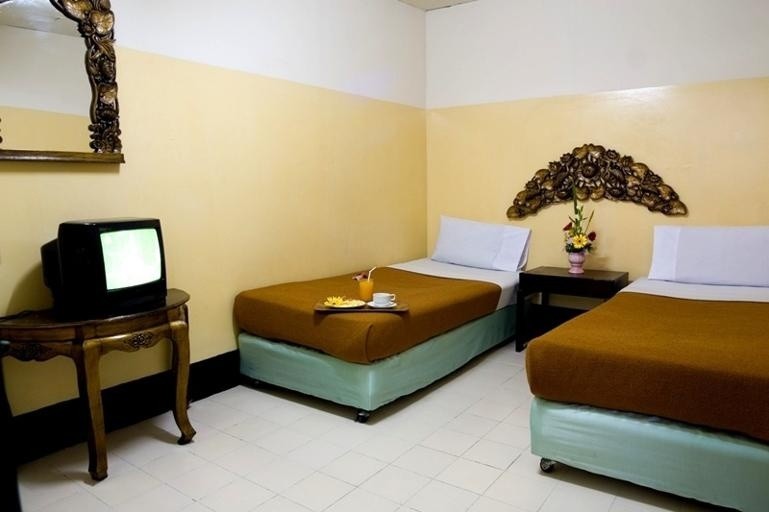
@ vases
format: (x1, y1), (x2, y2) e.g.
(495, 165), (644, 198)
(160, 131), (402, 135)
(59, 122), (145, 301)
(568, 247), (586, 274)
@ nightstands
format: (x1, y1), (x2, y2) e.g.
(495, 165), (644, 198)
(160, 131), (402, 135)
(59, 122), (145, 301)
(516, 265), (627, 351)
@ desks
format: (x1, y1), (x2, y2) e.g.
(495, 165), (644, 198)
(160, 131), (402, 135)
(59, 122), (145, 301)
(1, 286), (207, 484)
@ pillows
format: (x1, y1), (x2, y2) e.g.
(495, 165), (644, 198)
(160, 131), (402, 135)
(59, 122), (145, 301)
(431, 214), (529, 271)
(647, 223), (769, 287)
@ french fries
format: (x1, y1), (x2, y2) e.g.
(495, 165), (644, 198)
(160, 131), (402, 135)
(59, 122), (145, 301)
(325, 295), (347, 305)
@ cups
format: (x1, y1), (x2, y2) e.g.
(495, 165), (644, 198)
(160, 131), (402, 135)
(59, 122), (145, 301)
(358, 278), (374, 303)
(372, 292), (396, 306)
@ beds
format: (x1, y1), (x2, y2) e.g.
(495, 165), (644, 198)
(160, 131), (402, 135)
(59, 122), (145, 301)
(232, 257), (522, 426)
(524, 278), (769, 511)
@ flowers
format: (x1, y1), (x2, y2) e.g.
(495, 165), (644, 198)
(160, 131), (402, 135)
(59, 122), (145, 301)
(562, 203), (598, 250)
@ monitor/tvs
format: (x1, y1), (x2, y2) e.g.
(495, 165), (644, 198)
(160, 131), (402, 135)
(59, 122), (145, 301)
(40, 216), (166, 322)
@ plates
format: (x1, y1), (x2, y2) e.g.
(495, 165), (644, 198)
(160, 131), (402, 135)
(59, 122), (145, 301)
(367, 301), (398, 309)
(324, 299), (366, 308)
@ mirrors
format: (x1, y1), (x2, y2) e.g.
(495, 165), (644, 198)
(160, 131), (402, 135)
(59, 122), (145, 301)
(0, 1), (123, 161)
